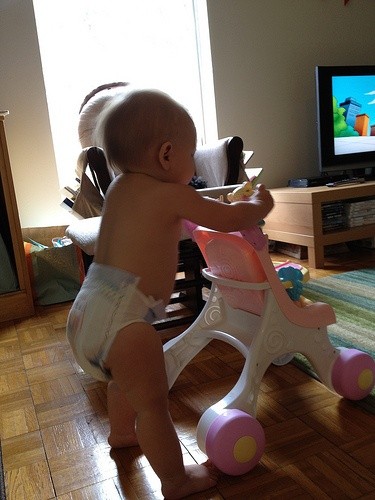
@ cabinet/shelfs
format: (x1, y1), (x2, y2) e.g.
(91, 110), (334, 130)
(260, 181), (375, 269)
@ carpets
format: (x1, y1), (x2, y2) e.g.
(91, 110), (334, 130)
(291, 270), (375, 397)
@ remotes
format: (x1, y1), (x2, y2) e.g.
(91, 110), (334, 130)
(326, 178), (365, 187)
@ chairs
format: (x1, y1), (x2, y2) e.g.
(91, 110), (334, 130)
(64, 83), (250, 335)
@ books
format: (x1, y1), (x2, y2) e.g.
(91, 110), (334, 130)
(58, 183), (80, 213)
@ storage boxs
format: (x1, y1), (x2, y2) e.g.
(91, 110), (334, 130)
(27, 238), (85, 308)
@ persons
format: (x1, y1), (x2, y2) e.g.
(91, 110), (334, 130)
(64, 88), (275, 499)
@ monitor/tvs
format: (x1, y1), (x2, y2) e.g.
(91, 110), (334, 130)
(314, 65), (375, 181)
